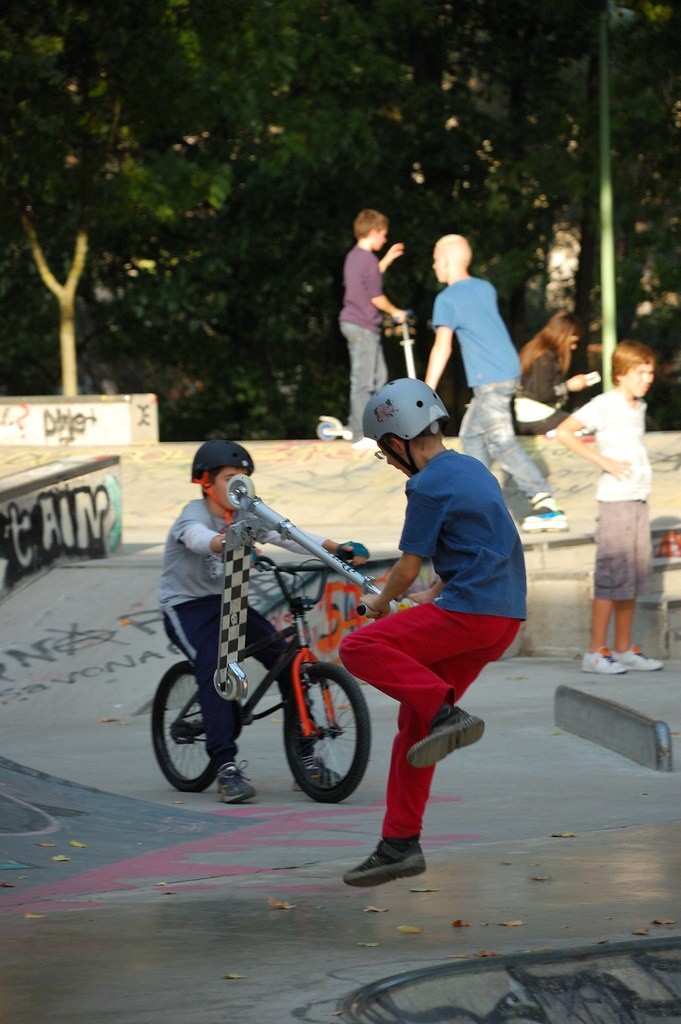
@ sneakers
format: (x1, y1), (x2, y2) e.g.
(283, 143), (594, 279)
(582, 646), (627, 674)
(612, 645), (663, 670)
(218, 759), (256, 803)
(292, 756), (340, 791)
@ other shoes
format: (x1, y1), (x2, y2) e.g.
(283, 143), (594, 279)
(343, 839), (426, 887)
(406, 706), (485, 767)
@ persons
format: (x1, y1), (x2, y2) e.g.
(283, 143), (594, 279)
(157, 440), (369, 804)
(557, 341), (664, 676)
(340, 210), (410, 450)
(422, 234), (570, 534)
(338, 377), (527, 889)
(512, 309), (592, 434)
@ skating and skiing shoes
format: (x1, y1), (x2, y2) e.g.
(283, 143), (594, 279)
(522, 492), (569, 533)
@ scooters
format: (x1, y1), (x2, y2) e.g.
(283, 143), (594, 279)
(316, 309), (418, 442)
(211, 473), (423, 703)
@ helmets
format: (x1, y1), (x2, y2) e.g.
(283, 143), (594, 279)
(363, 378), (450, 440)
(192, 439), (254, 482)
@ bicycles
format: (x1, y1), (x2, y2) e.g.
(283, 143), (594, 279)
(148, 559), (374, 805)
